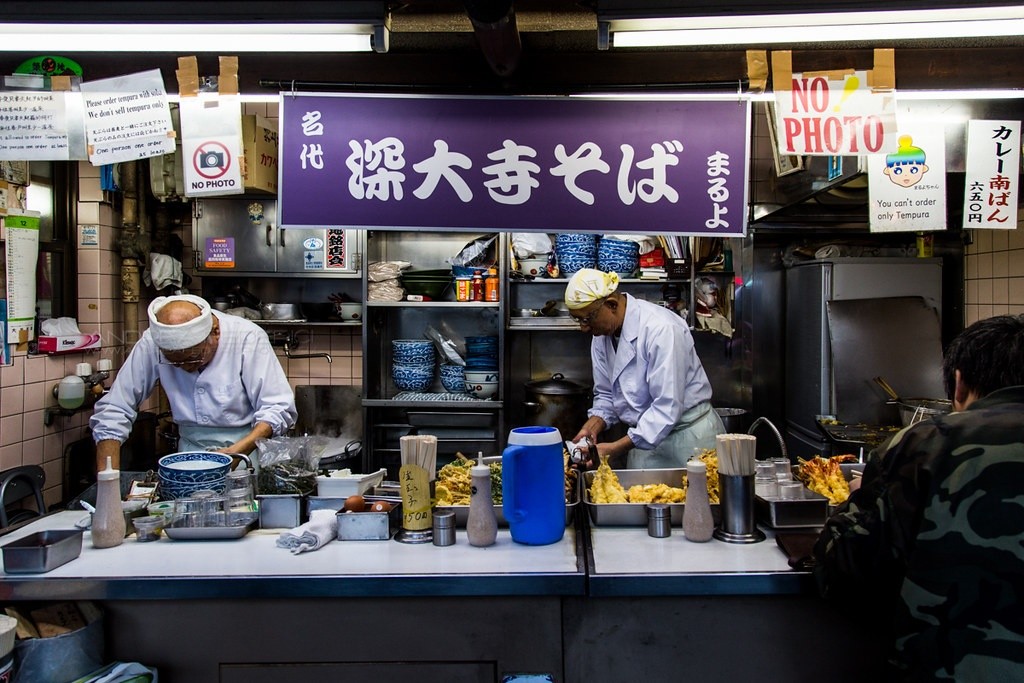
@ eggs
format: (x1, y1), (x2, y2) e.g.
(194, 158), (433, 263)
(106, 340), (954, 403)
(370, 501), (393, 511)
(344, 496), (365, 512)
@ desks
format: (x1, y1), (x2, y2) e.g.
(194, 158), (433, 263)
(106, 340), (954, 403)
(0, 508), (587, 683)
(586, 525), (813, 683)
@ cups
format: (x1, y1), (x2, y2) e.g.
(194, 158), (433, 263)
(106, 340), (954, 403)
(170, 470), (259, 528)
(712, 469), (767, 544)
(647, 503), (671, 538)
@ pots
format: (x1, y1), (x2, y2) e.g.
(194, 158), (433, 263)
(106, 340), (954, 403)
(12, 607), (109, 682)
(236, 285), (299, 320)
(520, 373), (591, 440)
(710, 408), (749, 434)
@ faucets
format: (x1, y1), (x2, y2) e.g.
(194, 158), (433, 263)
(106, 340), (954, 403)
(282, 342), (333, 363)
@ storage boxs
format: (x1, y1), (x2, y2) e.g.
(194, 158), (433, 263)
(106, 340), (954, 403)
(241, 113), (278, 199)
(335, 502), (400, 541)
(314, 467), (387, 496)
(254, 490), (315, 529)
(1, 529), (84, 574)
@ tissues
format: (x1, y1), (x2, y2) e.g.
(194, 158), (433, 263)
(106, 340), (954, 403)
(38, 316), (102, 352)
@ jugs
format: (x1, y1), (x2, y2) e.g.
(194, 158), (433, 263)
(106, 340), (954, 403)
(503, 425), (566, 545)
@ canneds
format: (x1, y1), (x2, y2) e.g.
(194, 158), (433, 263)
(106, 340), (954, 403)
(456, 278), (470, 302)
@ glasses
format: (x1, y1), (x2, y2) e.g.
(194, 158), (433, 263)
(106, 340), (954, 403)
(568, 298), (607, 324)
(158, 338), (209, 367)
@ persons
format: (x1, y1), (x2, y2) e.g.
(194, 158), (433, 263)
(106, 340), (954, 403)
(87, 294), (299, 471)
(811, 315), (1024, 682)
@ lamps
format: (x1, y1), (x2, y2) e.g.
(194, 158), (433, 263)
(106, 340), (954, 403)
(596, 1), (1024, 50)
(0, 4), (392, 54)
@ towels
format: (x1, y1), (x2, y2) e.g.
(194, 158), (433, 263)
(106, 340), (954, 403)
(142, 252), (183, 290)
(275, 508), (340, 556)
(602, 232), (656, 255)
(146, 295), (214, 350)
(221, 306), (262, 321)
(564, 267), (620, 310)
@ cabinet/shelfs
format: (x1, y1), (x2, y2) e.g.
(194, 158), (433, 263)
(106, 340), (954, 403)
(505, 231), (736, 336)
(360, 228), (503, 479)
(188, 195), (362, 325)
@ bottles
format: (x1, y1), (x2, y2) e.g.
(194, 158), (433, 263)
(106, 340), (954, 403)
(681, 446), (715, 542)
(466, 452), (498, 547)
(91, 455), (126, 548)
(469, 270), (483, 302)
(484, 269), (499, 302)
(754, 458), (807, 500)
(456, 278), (469, 302)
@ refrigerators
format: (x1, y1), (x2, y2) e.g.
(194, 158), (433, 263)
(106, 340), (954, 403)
(782, 258), (942, 462)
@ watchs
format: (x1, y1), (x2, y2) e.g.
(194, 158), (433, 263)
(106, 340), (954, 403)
(565, 268), (726, 468)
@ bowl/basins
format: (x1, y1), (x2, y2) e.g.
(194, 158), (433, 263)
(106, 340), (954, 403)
(158, 451), (234, 501)
(402, 265), (489, 299)
(335, 301), (362, 322)
(516, 233), (639, 279)
(393, 337), (498, 400)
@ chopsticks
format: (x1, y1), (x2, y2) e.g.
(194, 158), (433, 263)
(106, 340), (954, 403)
(715, 434), (756, 475)
